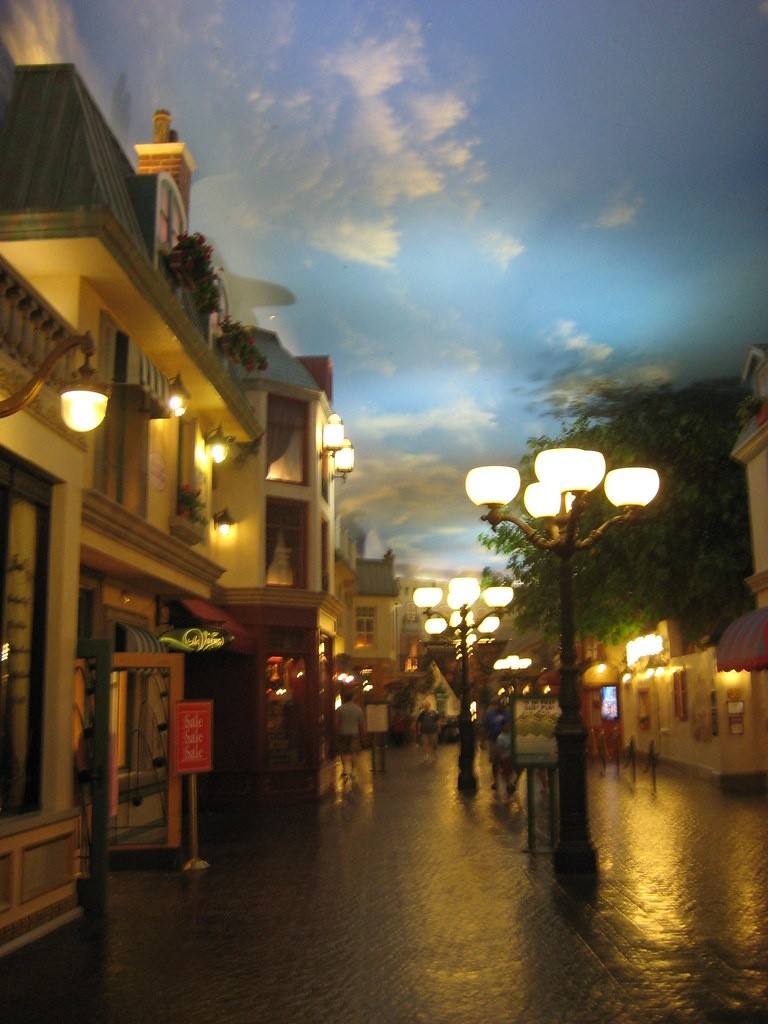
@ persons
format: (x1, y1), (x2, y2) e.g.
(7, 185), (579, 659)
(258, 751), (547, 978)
(390, 706), (405, 746)
(479, 696), (523, 797)
(415, 701), (442, 764)
(333, 692), (365, 777)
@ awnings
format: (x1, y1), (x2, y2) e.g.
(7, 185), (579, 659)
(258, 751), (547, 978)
(717, 607), (768, 672)
(166, 599), (255, 655)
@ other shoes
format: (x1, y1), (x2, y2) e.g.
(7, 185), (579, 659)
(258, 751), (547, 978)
(491, 783), (498, 789)
(507, 786), (515, 794)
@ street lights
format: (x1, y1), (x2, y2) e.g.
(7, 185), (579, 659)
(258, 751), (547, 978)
(465, 447), (661, 890)
(412, 576), (514, 796)
